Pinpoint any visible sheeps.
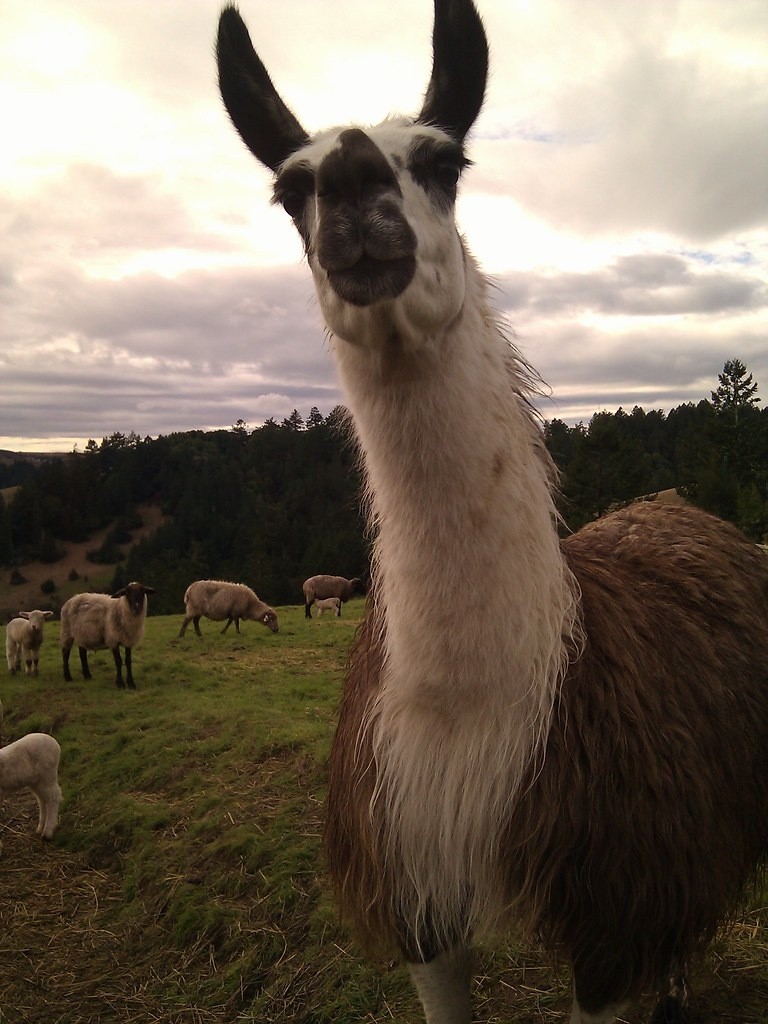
[60,581,157,690]
[178,580,280,638]
[0,733,62,842]
[304,575,365,618]
[6,610,54,676]
